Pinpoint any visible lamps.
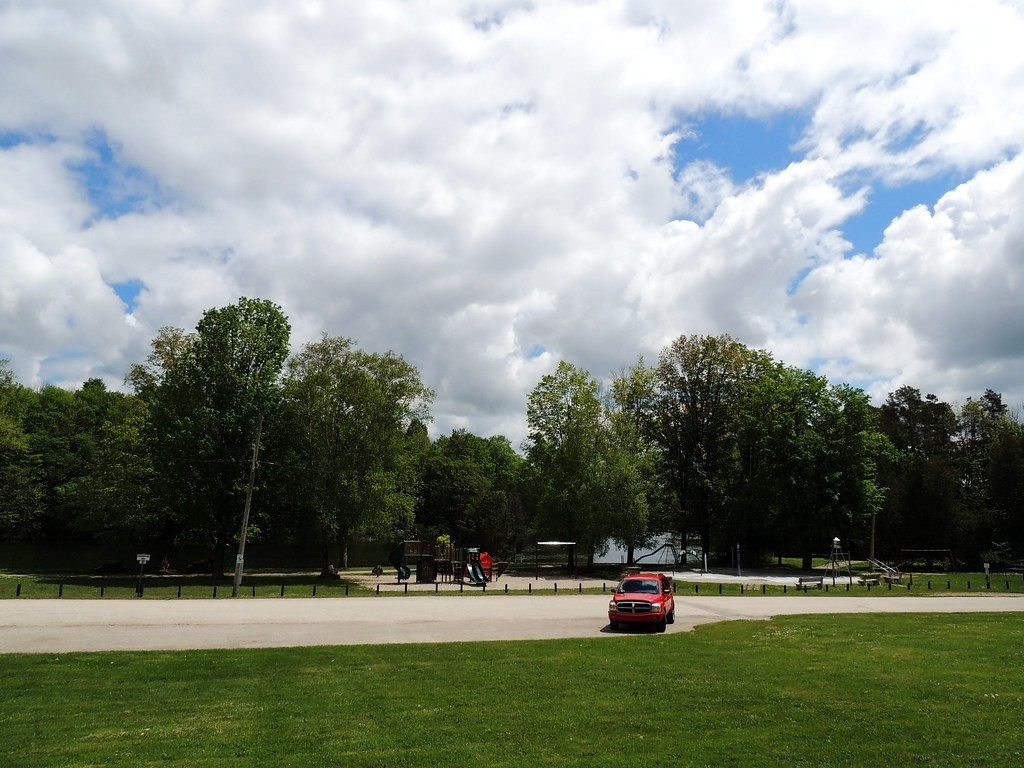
[984,563,990,568]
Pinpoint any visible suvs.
[608,573,675,632]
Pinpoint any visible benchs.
[884,572,904,583]
[858,574,881,586]
[536,564,578,580]
[794,576,824,590]
